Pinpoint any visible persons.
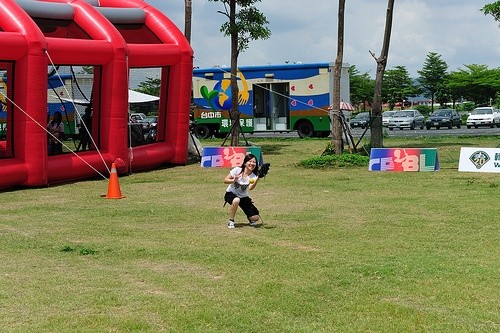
[224,154,270,228]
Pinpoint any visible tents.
[51,88,160,148]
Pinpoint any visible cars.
[466,107,500,128]
[350,112,371,129]
[379,111,397,127]
[139,116,157,132]
[426,109,462,129]
[388,110,425,131]
[131,113,146,121]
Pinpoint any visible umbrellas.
[328,99,355,111]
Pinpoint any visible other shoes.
[247,217,256,225]
[227,221,235,229]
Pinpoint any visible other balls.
[249,178,254,184]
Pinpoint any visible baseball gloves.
[257,163,271,178]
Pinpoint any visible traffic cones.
[101,163,126,199]
